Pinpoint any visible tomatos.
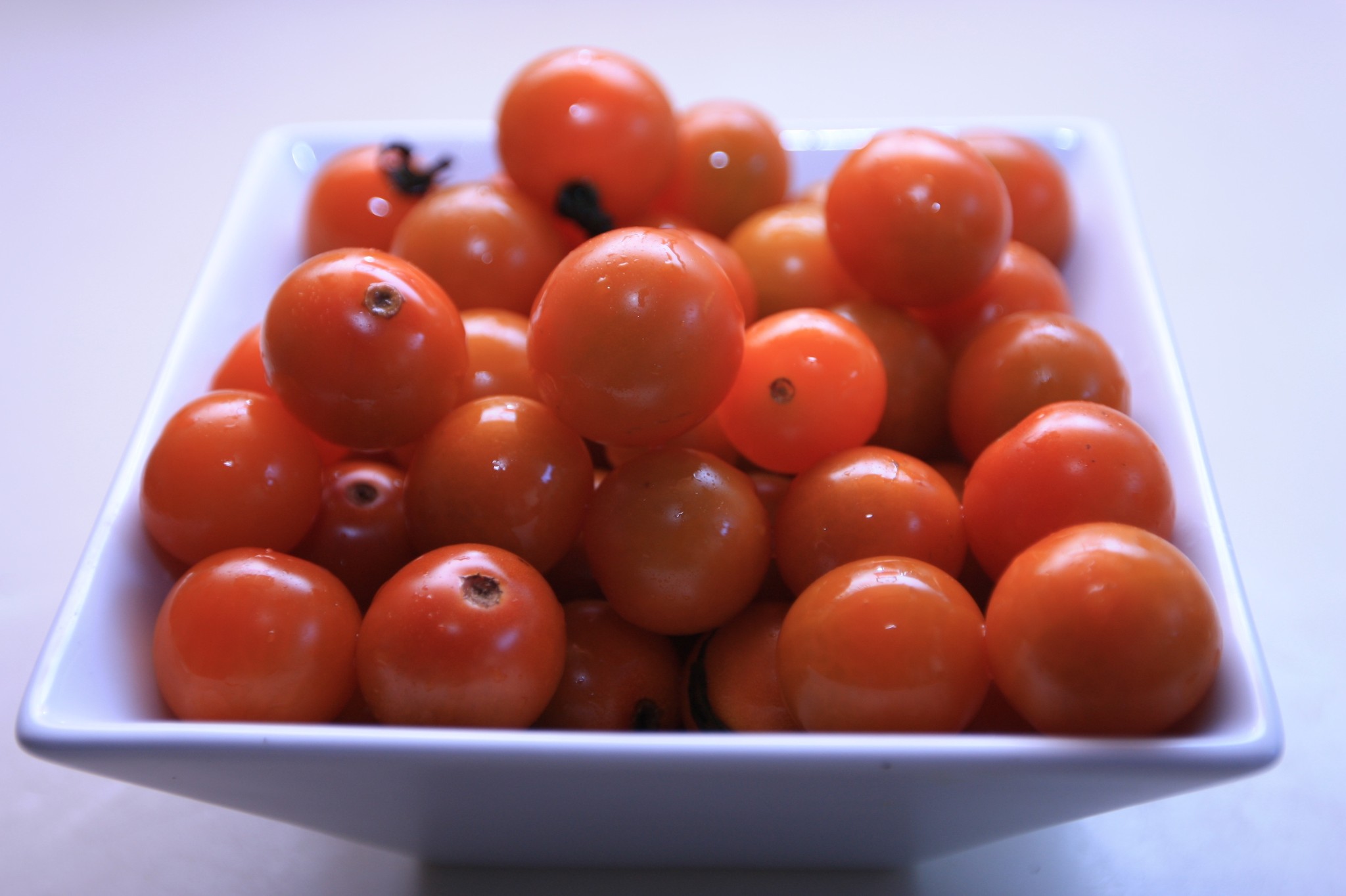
[137,43,1225,741]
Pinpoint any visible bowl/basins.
[14,119,1286,876]
